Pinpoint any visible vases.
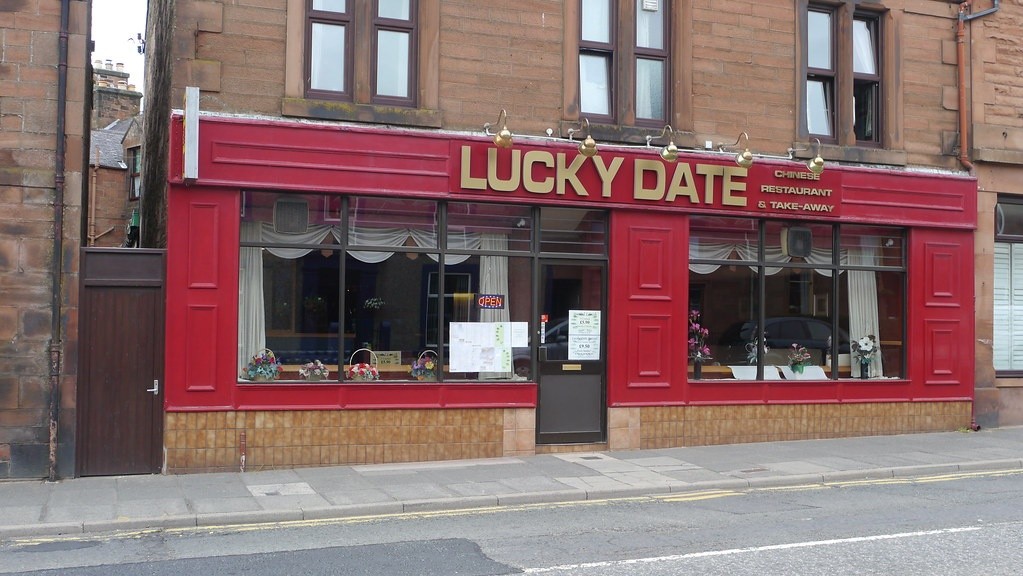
[694,361,701,380]
[861,362,869,379]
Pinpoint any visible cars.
[511,320,570,378]
[719,318,851,364]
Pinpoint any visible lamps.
[567,118,598,157]
[789,138,824,174]
[717,133,753,169]
[484,109,514,149]
[646,125,678,162]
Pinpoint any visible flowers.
[345,363,380,381]
[688,310,714,364]
[849,335,878,364]
[746,337,770,364]
[244,352,283,380]
[408,352,437,380]
[787,343,811,374]
[299,360,329,381]
